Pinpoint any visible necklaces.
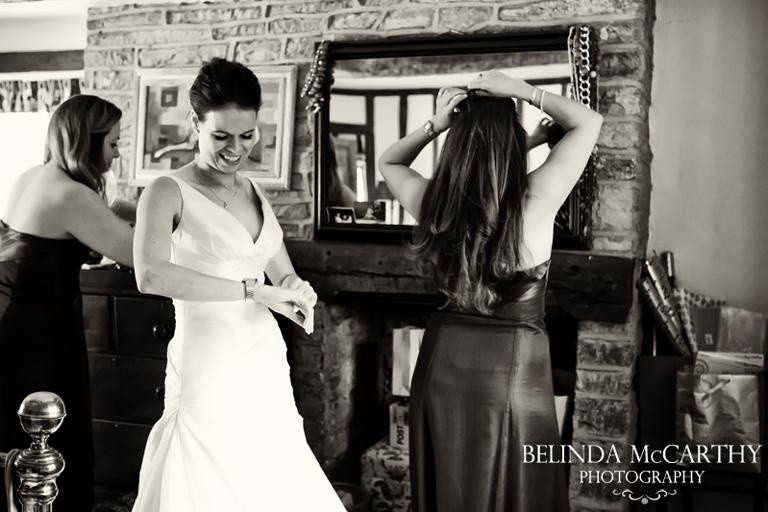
[194,163,240,209]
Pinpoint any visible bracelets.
[528,86,538,105]
[539,88,546,113]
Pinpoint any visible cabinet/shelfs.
[75,263,175,494]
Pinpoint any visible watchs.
[242,277,259,303]
[424,120,439,140]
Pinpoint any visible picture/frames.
[127,64,298,192]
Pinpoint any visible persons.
[0,94,134,511]
[134,58,349,511]
[377,72,606,511]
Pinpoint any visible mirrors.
[311,23,597,253]
